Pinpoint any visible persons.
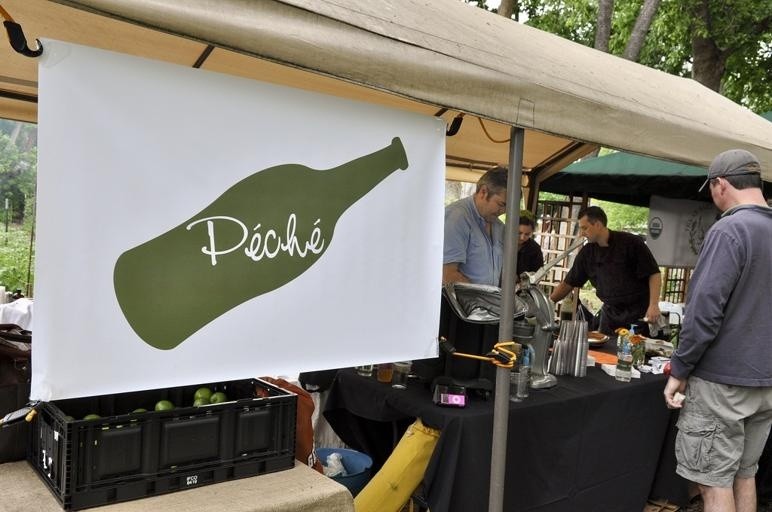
[663,148,772,512]
[548,206,663,340]
[440,165,522,287]
[516,216,544,285]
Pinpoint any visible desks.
[322,341,702,512]
[0,457,354,512]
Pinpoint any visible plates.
[587,331,610,344]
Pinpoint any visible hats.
[698,149,760,193]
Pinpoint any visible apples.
[66,388,226,430]
[619,328,630,336]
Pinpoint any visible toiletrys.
[622,324,639,353]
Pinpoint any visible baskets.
[26,379,299,512]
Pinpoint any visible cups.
[548,320,588,378]
[391,361,413,390]
[509,366,533,404]
[376,363,394,384]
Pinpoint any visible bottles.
[614,342,634,382]
